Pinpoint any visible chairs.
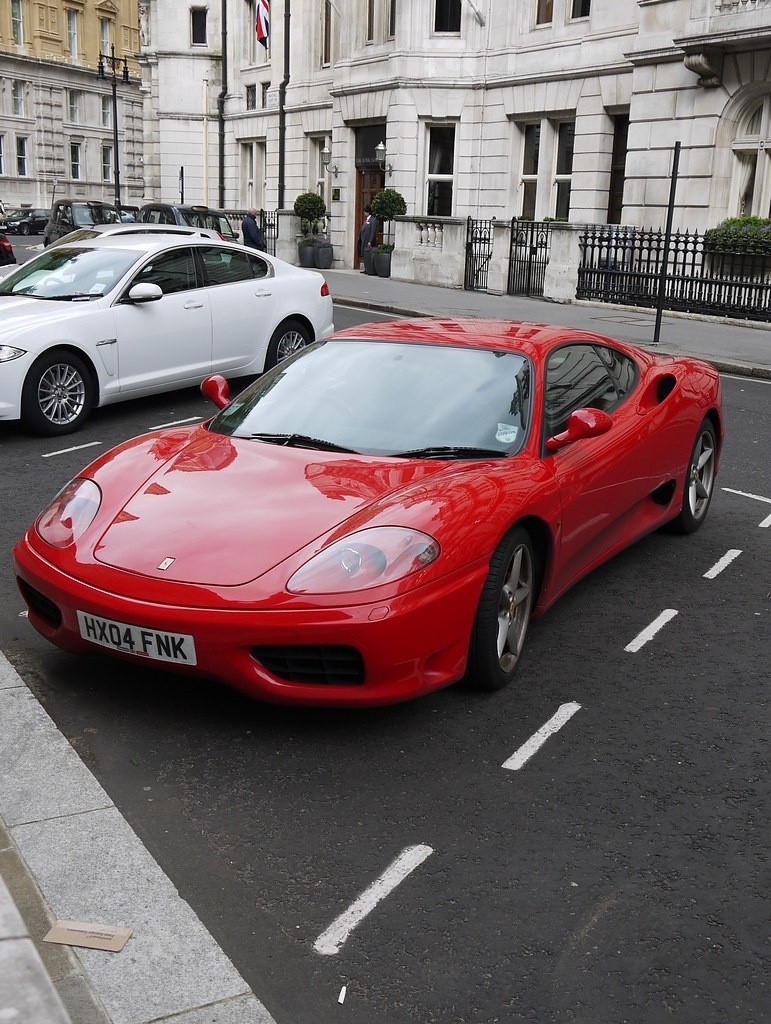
[224,255,251,284]
[204,252,226,283]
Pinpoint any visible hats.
[363,205,372,211]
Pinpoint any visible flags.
[256,0,269,50]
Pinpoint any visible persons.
[358,207,377,274]
[242,208,264,252]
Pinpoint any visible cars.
[43,200,123,247]
[0,223,232,294]
[135,203,240,245]
[0,209,51,236]
[106,210,135,223]
[0,233,334,438]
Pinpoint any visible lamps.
[320,147,338,178]
[374,140,392,177]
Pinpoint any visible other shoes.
[360,270,367,274]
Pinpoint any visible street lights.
[96,43,132,206]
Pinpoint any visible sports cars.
[13,316,725,709]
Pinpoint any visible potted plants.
[363,246,379,275]
[373,243,394,278]
[313,241,333,269]
[298,237,320,268]
